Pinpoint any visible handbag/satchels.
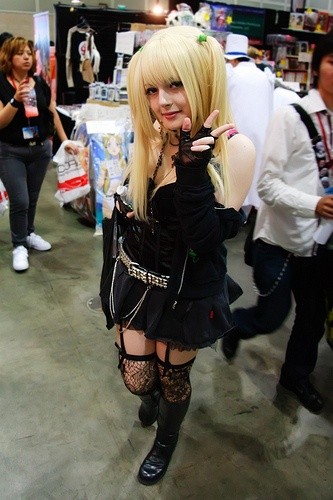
[53,139,91,203]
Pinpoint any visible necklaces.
[151,129,180,182]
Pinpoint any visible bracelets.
[9,97,21,108]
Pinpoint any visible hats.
[224,34,255,60]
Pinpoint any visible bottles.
[21,79,38,118]
[117,184,133,210]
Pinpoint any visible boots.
[139,427,181,486]
[138,398,159,427]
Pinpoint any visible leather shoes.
[279,373,324,413]
[221,307,240,360]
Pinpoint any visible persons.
[100,25,255,484]
[0,37,77,272]
[0,31,13,51]
[205,34,333,412]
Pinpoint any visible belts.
[119,242,169,289]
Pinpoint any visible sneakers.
[12,245,30,271]
[26,232,52,251]
